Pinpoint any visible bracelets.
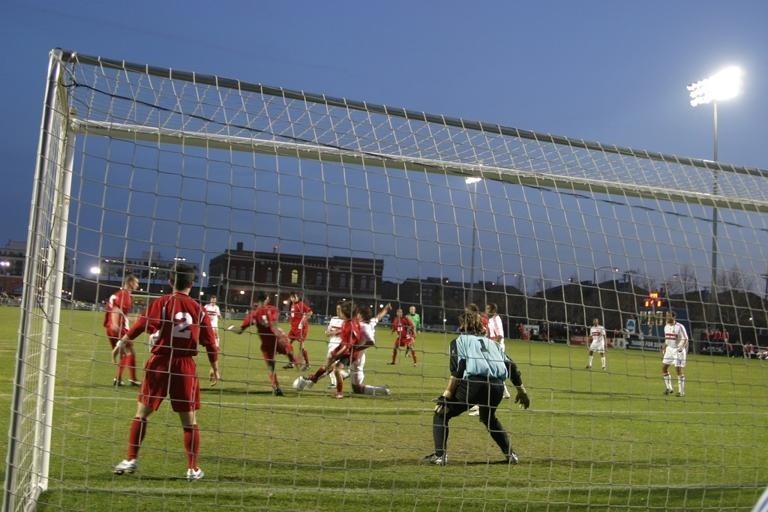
[115,339,127,349]
[514,384,527,394]
[441,389,454,400]
[227,325,235,330]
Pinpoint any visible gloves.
[432,395,451,415]
[515,393,530,409]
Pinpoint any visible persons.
[355,302,392,398]
[280,289,314,372]
[584,317,607,371]
[324,301,350,390]
[699,326,768,360]
[419,312,531,466]
[298,300,363,399]
[102,274,142,389]
[402,305,421,362]
[659,310,689,397]
[386,307,418,368]
[225,291,303,398]
[484,302,511,400]
[465,303,489,418]
[110,260,221,484]
[205,295,224,351]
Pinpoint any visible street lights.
[686,66,746,287]
[594,265,618,321]
[465,160,487,308]
[494,272,519,302]
[672,272,699,293]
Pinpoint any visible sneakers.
[423,454,447,464]
[113,378,141,386]
[283,363,295,368]
[664,389,685,397]
[187,467,204,481]
[507,453,518,464]
[301,375,350,400]
[469,406,479,416]
[302,366,311,371]
[274,388,283,396]
[383,385,391,397]
[114,459,136,474]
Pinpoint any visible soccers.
[292,378,305,390]
